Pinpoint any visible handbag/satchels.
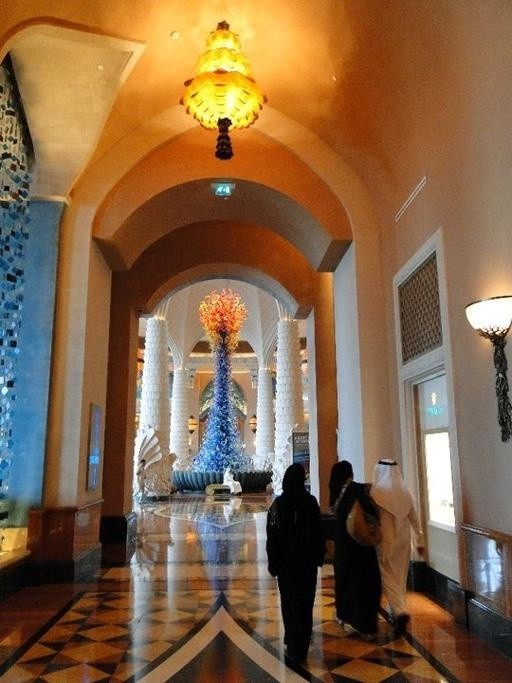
[346,483,383,546]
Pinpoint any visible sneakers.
[394,614,409,638]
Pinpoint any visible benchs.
[205,483,243,498]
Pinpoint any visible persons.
[265,462,326,663]
[134,458,147,505]
[327,460,381,641]
[369,456,425,635]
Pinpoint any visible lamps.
[178,19,269,162]
[464,293,512,443]
[248,414,257,432]
[188,414,197,434]
